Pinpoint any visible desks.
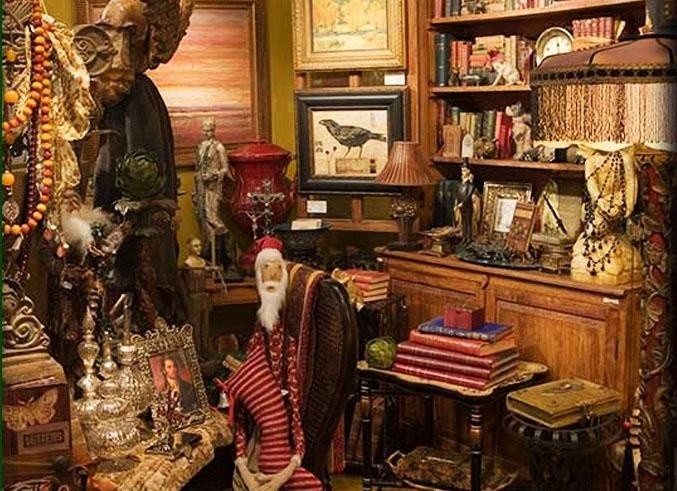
[95,411,234,491]
[354,358,548,491]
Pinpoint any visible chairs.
[179,277,360,490]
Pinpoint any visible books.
[342,268,391,302]
[345,393,385,461]
[435,33,532,86]
[571,16,629,43]
[506,375,625,430]
[434,0,555,18]
[391,315,521,391]
[450,105,513,159]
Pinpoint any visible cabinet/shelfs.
[382,0,677,491]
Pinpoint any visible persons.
[159,353,196,412]
[252,237,290,331]
[191,117,230,239]
[453,156,476,246]
[183,237,214,356]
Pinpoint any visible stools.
[502,412,623,491]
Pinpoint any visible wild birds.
[318,118,387,158]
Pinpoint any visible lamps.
[375,140,439,252]
[530,27,677,489]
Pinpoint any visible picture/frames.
[290,0,409,74]
[75,0,272,168]
[130,317,212,436]
[292,85,411,197]
[479,179,533,236]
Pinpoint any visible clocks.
[535,26,575,67]
